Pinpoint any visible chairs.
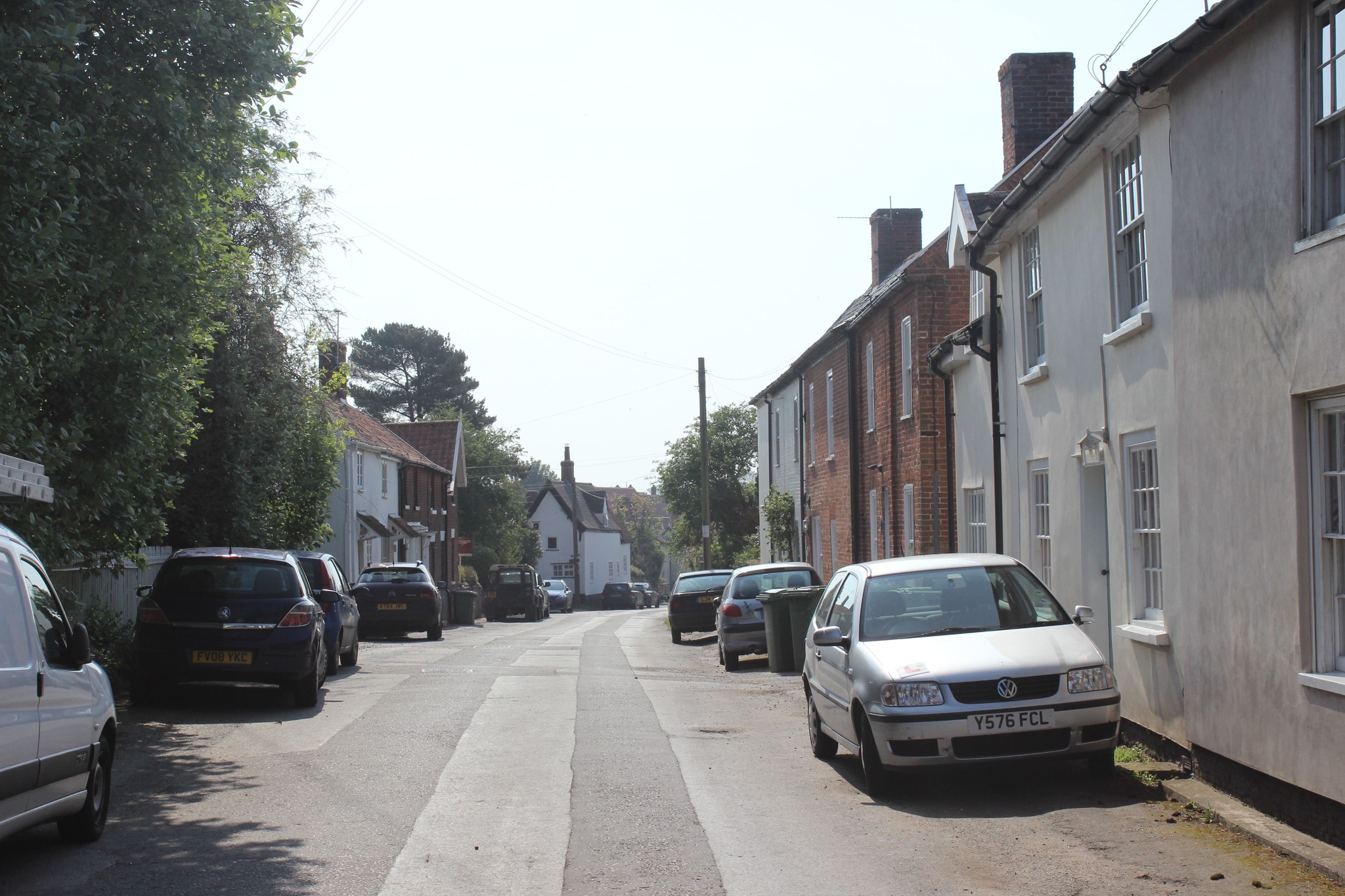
[862,591,908,637]
[739,581,761,599]
[787,574,807,590]
[954,581,1000,632]
[252,569,285,598]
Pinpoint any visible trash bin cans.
[451,589,482,626]
[754,587,795,672]
[783,585,829,675]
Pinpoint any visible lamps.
[1079,426,1109,468]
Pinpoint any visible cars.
[660,569,735,643]
[711,561,825,672]
[801,551,1123,796]
[542,579,573,613]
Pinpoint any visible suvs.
[276,549,371,676]
[633,582,660,608]
[131,546,329,708]
[484,563,542,622]
[499,573,551,618]
[348,559,446,641]
[600,581,644,609]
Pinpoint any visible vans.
[0,521,117,841]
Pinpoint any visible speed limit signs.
[696,553,704,564]
